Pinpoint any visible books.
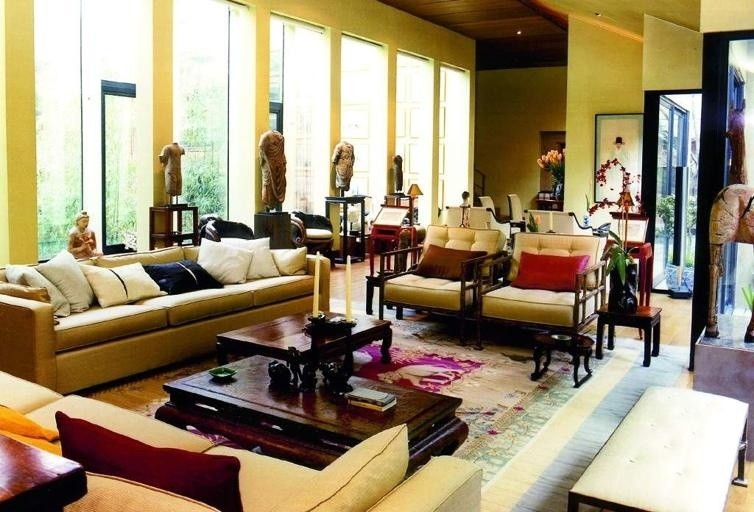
[348,387,397,412]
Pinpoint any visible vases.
[553,179,564,201]
[608,270,638,315]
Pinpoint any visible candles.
[312,250,320,318]
[345,255,352,323]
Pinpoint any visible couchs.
[378,227,607,336]
[1,248,330,395]
[0,371,483,511]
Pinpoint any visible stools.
[530,333,595,388]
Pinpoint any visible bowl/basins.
[208,367,237,382]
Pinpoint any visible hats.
[616,137,622,143]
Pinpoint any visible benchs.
[568,384,748,512]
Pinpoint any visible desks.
[595,303,662,369]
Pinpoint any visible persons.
[67,211,97,258]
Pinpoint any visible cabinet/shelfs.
[150,193,413,268]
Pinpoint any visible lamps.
[407,184,424,196]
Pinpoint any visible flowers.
[537,146,565,183]
[588,159,646,287]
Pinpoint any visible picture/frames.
[594,113,644,203]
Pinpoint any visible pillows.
[57,408,245,511]
[0,402,59,443]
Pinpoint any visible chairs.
[445,194,576,242]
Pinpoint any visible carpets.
[95,297,693,512]
